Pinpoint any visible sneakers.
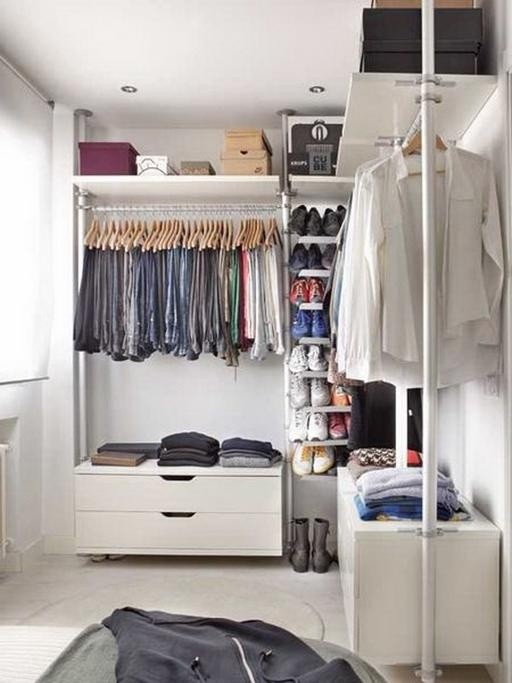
[289,345,328,374]
[291,242,336,270]
[289,205,346,236]
[290,413,352,442]
[291,310,328,338]
[292,445,349,476]
[291,377,353,408]
[290,277,325,305]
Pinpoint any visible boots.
[312,518,331,572]
[290,518,309,573]
